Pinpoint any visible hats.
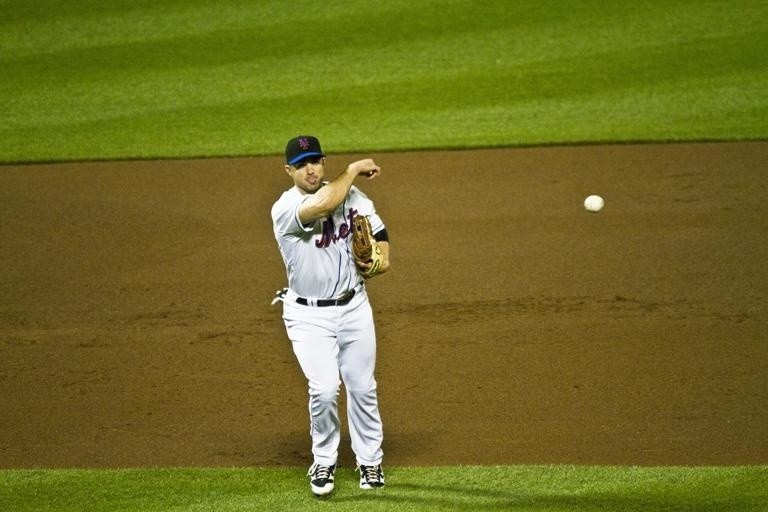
[285,135,324,166]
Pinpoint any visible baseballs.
[584,195,603,211]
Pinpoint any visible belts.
[297,281,366,306]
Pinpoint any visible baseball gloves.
[351,215,383,280]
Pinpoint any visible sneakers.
[355,460,385,491]
[308,460,338,496]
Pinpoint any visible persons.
[270,135,390,496]
